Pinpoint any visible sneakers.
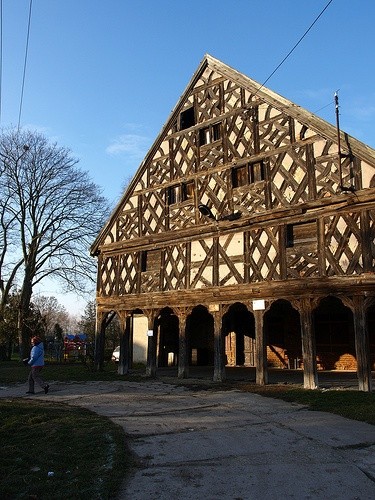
[25,391,34,394]
[44,385,50,394]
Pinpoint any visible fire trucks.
[63,334,87,363]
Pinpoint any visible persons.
[23,335,50,395]
